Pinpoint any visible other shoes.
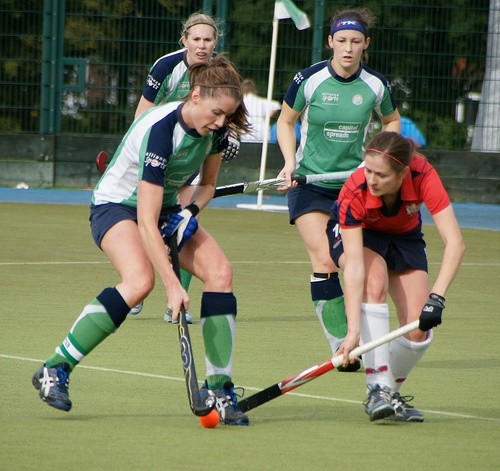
[128,300,143,315]
[335,346,360,373]
[164,308,194,324]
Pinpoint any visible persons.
[275,7,401,372]
[325,132,465,423]
[32,59,251,426]
[269,120,301,143]
[238,79,281,143]
[129,12,240,323]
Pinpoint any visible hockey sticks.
[200,319,420,413]
[95,151,358,199]
[168,229,217,416]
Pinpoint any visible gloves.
[162,203,199,256]
[419,293,445,332]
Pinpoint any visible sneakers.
[384,392,424,422]
[363,383,394,422]
[199,380,249,426]
[32,361,72,412]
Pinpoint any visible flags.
[274,0,310,30]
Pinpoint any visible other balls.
[200,409,220,429]
